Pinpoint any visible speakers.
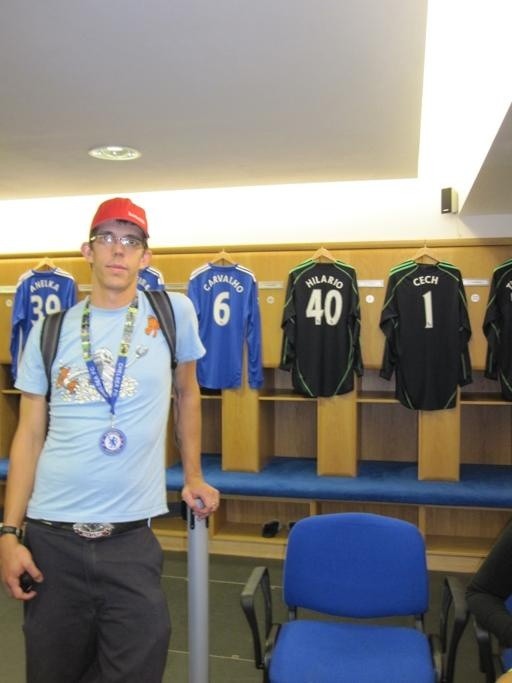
[440,187,458,214]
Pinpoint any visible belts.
[42,515,148,538]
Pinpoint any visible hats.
[89,196,147,237]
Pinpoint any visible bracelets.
[0,522,22,539]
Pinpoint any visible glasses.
[89,233,142,248]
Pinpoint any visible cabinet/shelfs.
[0,236,510,575]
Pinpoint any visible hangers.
[18,255,76,285]
[187,244,461,284]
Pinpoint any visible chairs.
[241,512,472,683]
[449,512,511,683]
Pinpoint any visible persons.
[0,196,219,683]
[465,518,511,682]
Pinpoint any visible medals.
[97,425,129,455]
